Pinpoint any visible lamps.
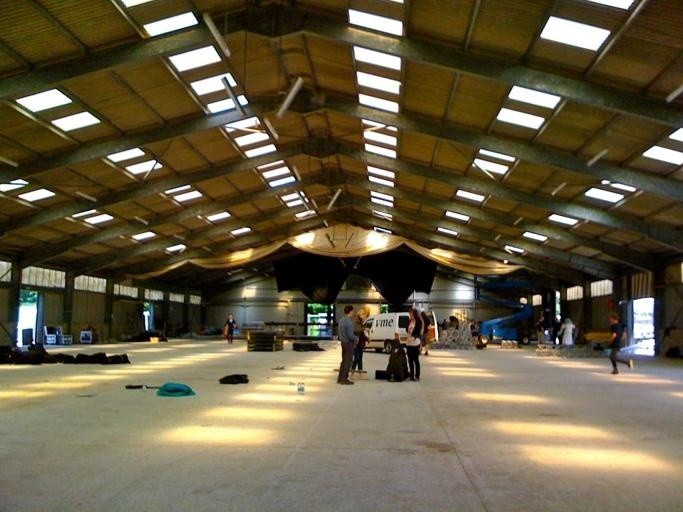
[201,11,307,140]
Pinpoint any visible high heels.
[408,375,421,381]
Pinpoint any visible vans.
[362,311,439,353]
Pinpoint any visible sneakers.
[351,370,369,380]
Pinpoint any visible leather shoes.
[337,378,355,385]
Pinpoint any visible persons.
[536,316,545,346]
[336,305,357,384]
[405,308,424,382]
[351,309,371,373]
[468,318,476,338]
[557,317,576,359]
[419,311,430,355]
[550,318,562,349]
[608,313,634,375]
[441,319,449,330]
[224,314,237,345]
